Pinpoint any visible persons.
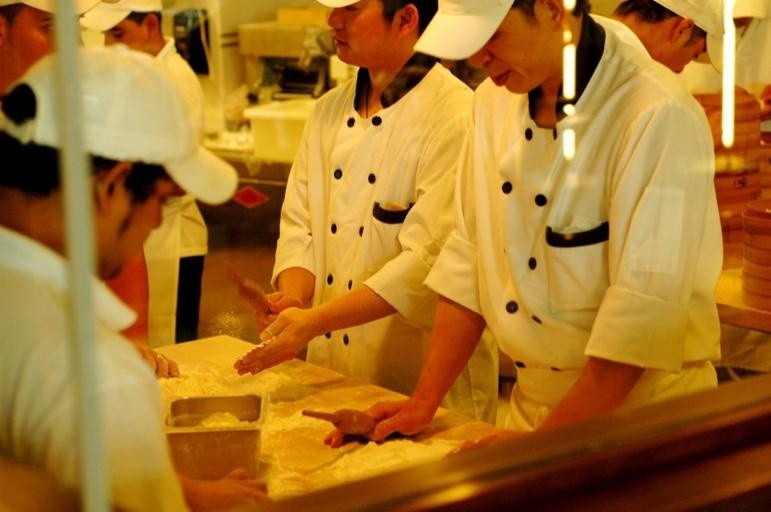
[232,0,498,427]
[1,1,182,381]
[0,40,275,512]
[79,0,210,345]
[611,0,722,74]
[324,0,724,462]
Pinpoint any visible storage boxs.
[243,97,319,161]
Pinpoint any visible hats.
[78,0,166,32]
[1,0,102,20]
[411,1,517,62]
[1,42,243,210]
[653,1,726,76]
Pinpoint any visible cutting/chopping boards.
[153,334,502,500]
